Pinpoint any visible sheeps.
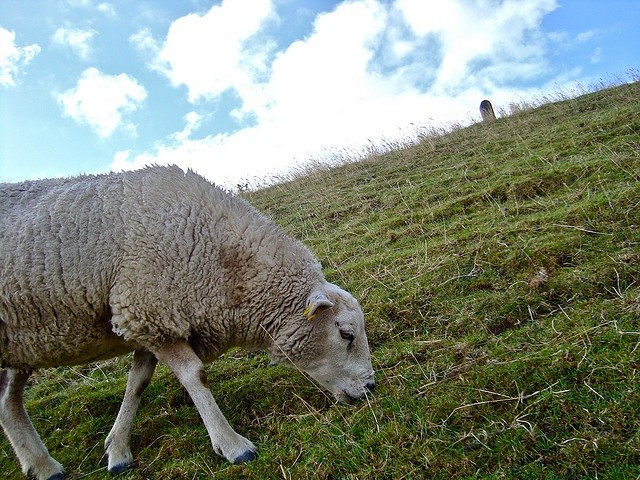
[0,164,376,480]
